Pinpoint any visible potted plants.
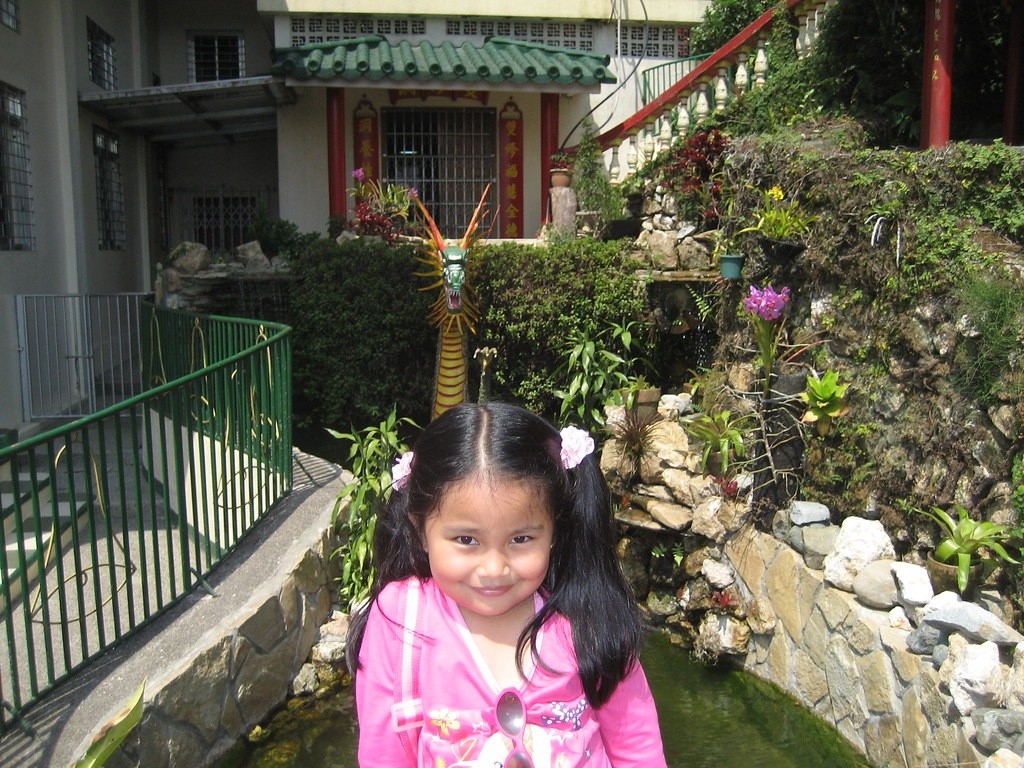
[720,241,745,280]
[736,186,820,268]
[752,362,850,532]
[602,372,661,425]
[912,503,1023,601]
[680,411,759,481]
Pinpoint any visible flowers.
[560,426,595,470]
[550,153,573,168]
[392,451,414,491]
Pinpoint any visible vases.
[550,168,569,187]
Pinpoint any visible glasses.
[495,686,535,768]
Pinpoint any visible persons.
[356,402,669,768]
